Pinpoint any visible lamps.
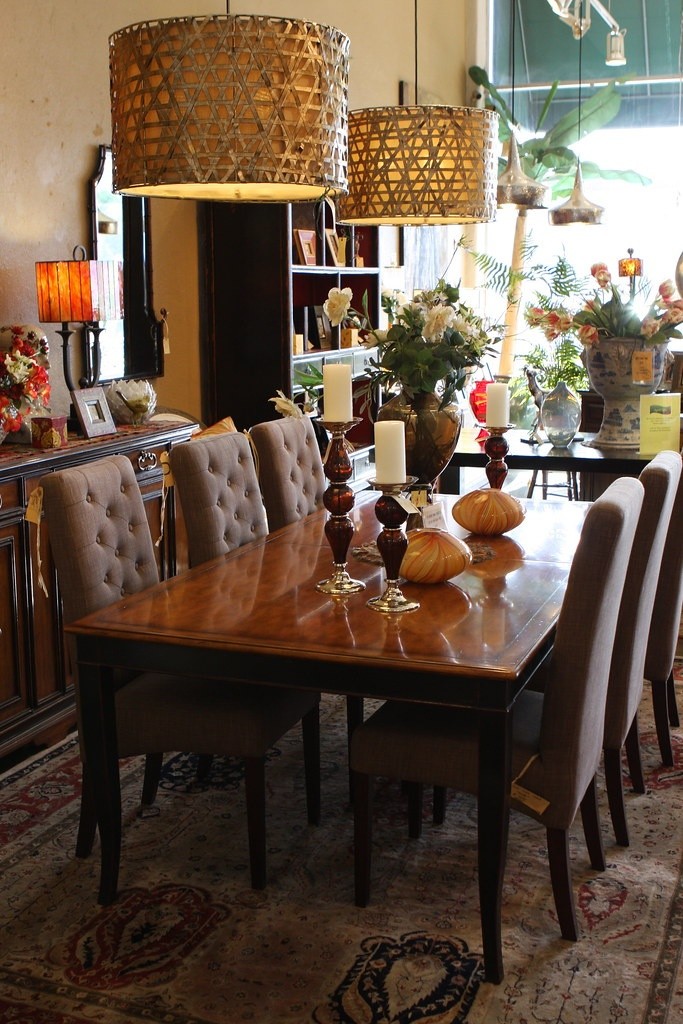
[108,0,350,203]
[337,0,501,227]
[547,0,627,66]
[549,0,604,226]
[496,0,550,211]
[618,248,643,296]
[35,244,124,432]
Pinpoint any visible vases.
[586,335,670,449]
[540,383,581,448]
[0,398,19,444]
[378,384,463,505]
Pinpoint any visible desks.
[62,493,596,984]
[368,425,683,501]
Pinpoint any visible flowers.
[526,263,683,349]
[0,322,52,432]
[324,230,535,467]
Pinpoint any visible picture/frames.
[297,306,332,353]
[71,386,118,438]
[293,229,317,266]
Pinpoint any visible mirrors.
[86,146,168,388]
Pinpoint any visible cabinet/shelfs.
[0,421,199,776]
[215,200,384,489]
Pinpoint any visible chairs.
[250,416,326,531]
[168,433,369,800]
[353,451,682,940]
[38,456,320,893]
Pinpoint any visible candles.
[322,362,353,421]
[373,421,406,485]
[487,383,510,428]
